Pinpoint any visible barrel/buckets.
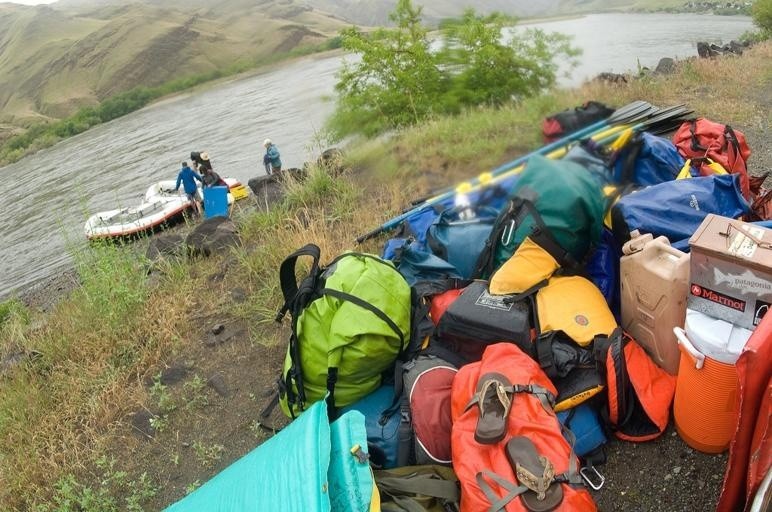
[202,185,228,222]
[617,228,692,377]
[446,215,496,279]
[672,309,753,457]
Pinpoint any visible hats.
[200,152,208,160]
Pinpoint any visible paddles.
[356,100,696,243]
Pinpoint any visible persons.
[263,139,282,173]
[190,151,212,174]
[198,166,231,194]
[174,162,203,216]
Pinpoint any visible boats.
[83,198,195,245]
[143,176,250,210]
